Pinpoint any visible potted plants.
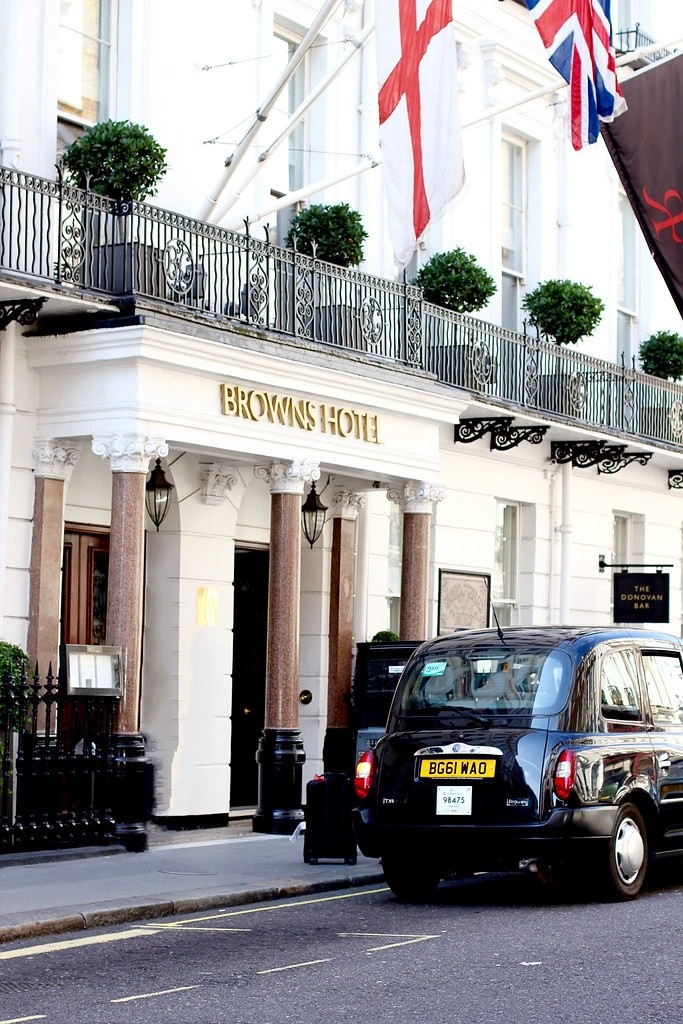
[286,203,367,353]
[638,330,683,444]
[59,118,168,301]
[409,248,492,391]
[520,277,604,419]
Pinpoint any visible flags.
[372,0,465,275]
[525,0,628,151]
[598,51,683,319]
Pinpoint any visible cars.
[353,624,683,900]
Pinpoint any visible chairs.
[419,666,543,701]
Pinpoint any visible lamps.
[144,458,175,532]
[300,474,331,549]
